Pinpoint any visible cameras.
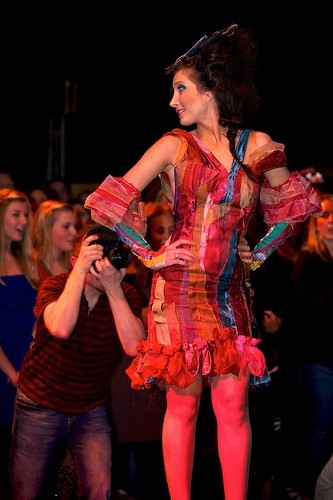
[89,233,132,273]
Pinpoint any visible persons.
[83,25,322,500]
[11,226,145,500]
[0,170,333,500]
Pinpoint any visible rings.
[175,253,179,258]
[248,253,253,258]
